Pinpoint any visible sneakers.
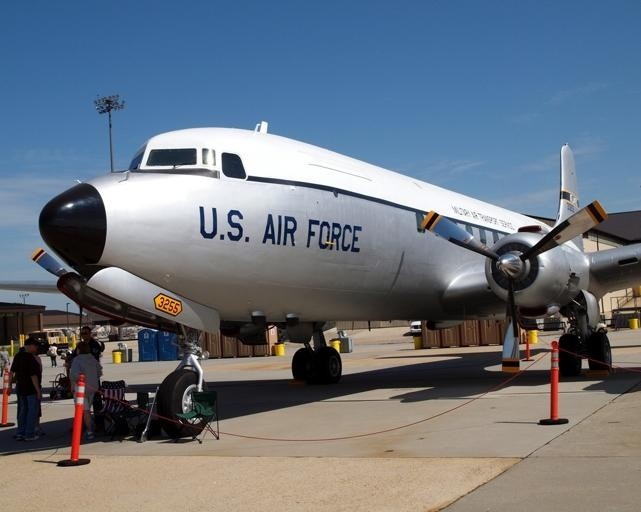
[82,433,95,440]
[15,429,45,441]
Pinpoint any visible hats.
[25,338,40,345]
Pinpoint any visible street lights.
[90,94,126,175]
[65,301,70,330]
[17,293,29,305]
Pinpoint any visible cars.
[25,328,68,353]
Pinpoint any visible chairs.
[176,391,219,444]
[98,380,127,435]
[109,392,149,439]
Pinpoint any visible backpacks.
[50,350,57,357]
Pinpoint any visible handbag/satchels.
[47,351,50,356]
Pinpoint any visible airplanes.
[27,113,641,439]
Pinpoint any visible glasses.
[81,332,89,335]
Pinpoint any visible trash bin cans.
[329,339,341,354]
[528,330,537,343]
[112,350,122,363]
[628,318,638,329]
[274,343,285,356]
[413,332,422,349]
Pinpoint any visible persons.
[64,326,105,440]
[7,339,43,441]
[0,345,10,377]
[48,343,58,368]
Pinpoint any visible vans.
[408,320,421,335]
